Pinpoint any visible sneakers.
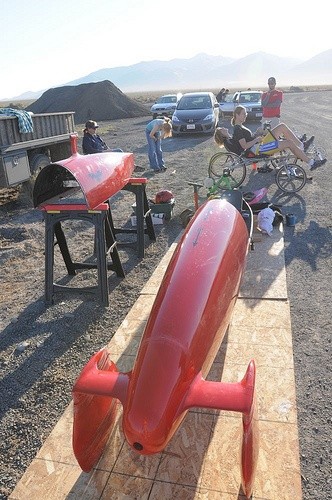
[303,136,315,152]
[310,159,327,170]
[301,133,307,142]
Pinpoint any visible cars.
[150,94,181,119]
[232,90,267,122]
[171,92,221,137]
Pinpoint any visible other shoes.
[154,167,161,171]
[160,165,167,170]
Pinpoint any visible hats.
[268,77,276,85]
[85,120,99,128]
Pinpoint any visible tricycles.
[206,120,328,195]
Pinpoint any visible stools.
[131,199,176,219]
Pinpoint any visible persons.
[146,117,172,172]
[215,106,327,171]
[82,120,123,154]
[258,77,282,173]
[216,88,229,103]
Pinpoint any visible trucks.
[0,112,77,191]
